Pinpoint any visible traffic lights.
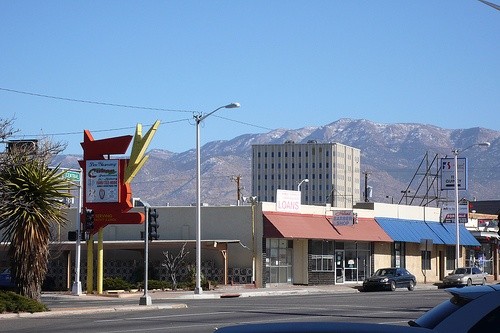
[84,207,94,231]
[68,231,78,241]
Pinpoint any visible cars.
[408,281,500,333]
[363,267,416,292]
[442,267,488,288]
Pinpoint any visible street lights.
[297,178,309,191]
[453,141,491,271]
[194,102,240,294]
